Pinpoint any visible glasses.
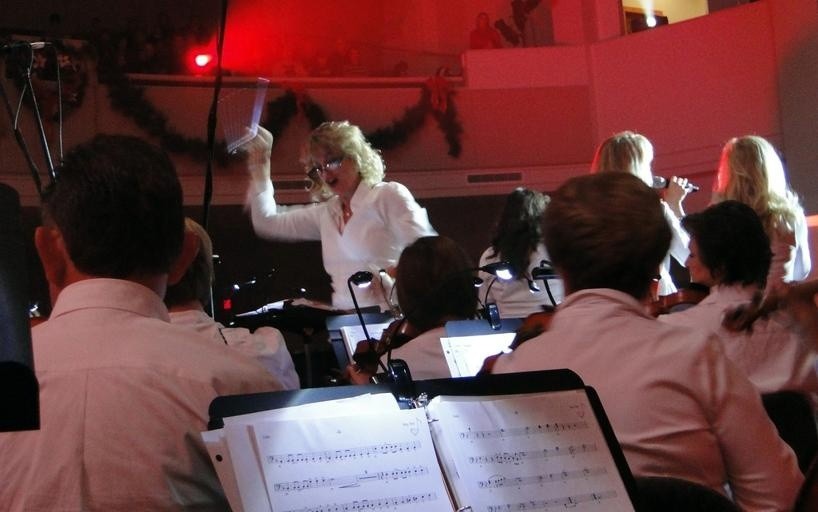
[306,156,344,180]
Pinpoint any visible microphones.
[651,175,702,193]
[2,40,51,54]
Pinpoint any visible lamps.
[222,261,561,382]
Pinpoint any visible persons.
[243,121,438,369]
[346,237,494,382]
[710,135,812,284]
[490,173,804,512]
[1,133,299,511]
[656,201,818,409]
[479,189,564,321]
[469,15,501,49]
[164,216,300,393]
[591,132,694,301]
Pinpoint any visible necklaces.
[340,207,354,219]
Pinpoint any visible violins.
[476,305,555,376]
[650,286,709,319]
[352,318,419,373]
